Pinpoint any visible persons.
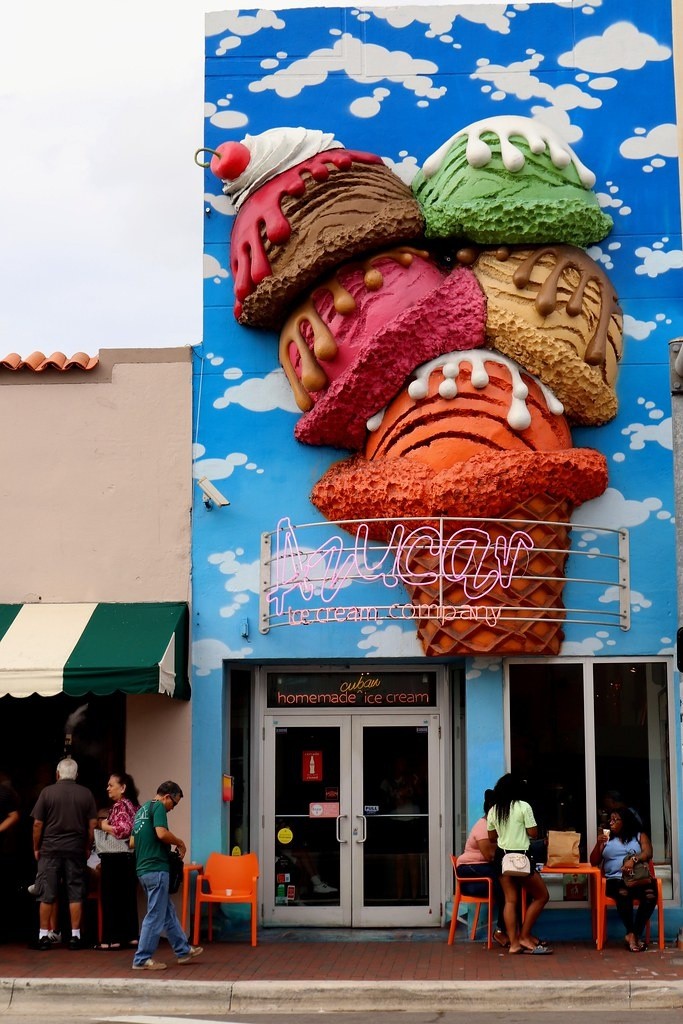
[590,805,658,952]
[0,783,20,832]
[30,758,113,951]
[94,772,142,950]
[487,774,553,955]
[602,783,651,844]
[386,757,424,900]
[456,789,547,947]
[277,817,338,893]
[130,780,204,971]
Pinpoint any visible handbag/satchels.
[623,848,649,882]
[93,801,135,854]
[547,830,580,868]
[502,854,530,876]
[166,850,183,894]
[276,856,294,874]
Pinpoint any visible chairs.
[86,864,107,943]
[193,853,259,947]
[598,860,665,950]
[447,855,520,950]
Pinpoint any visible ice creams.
[195,114,624,656]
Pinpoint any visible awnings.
[0,601,191,701]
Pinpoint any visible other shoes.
[624,935,639,952]
[46,930,62,942]
[125,938,138,949]
[37,935,52,950]
[94,940,121,951]
[68,936,84,950]
[636,940,648,951]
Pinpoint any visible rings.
[628,869,629,870]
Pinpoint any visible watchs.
[632,857,638,863]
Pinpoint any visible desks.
[522,863,607,942]
[181,864,204,933]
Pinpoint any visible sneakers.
[177,946,203,963]
[314,883,338,893]
[131,959,168,970]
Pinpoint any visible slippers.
[509,946,527,955]
[493,934,511,948]
[535,940,547,947]
[523,945,554,954]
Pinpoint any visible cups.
[226,889,232,896]
[603,829,610,844]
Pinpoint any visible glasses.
[168,795,177,806]
[608,817,622,823]
